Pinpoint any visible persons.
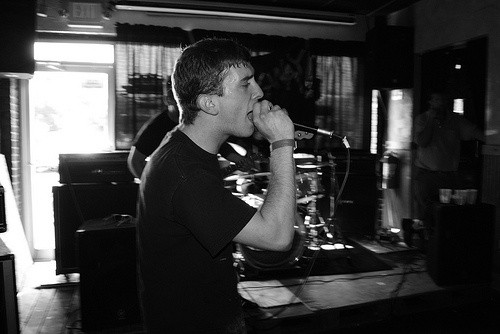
[128,76,180,180]
[137,40,296,334]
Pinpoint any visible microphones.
[291,121,346,141]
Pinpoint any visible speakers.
[54,182,140,274]
[74,215,142,334]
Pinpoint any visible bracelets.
[270,139,297,150]
